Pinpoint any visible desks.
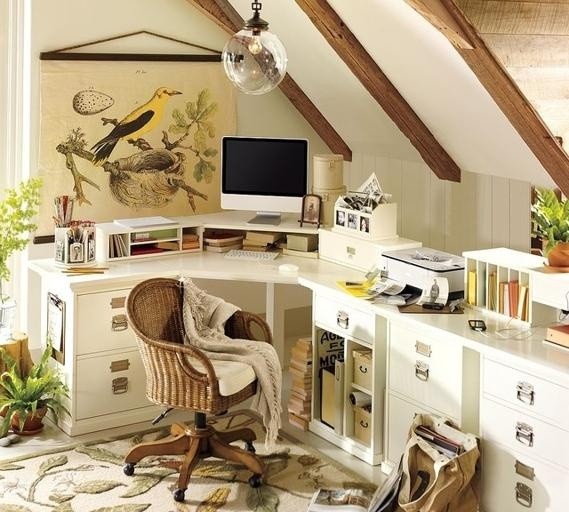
[27,210,568,511]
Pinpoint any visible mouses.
[278,263,300,272]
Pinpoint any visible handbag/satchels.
[395,414,482,512]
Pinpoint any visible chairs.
[123,279,275,500]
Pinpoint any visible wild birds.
[89,87,183,168]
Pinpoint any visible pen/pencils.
[346,282,362,285]
[62,268,109,276]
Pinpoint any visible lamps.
[221,0,289,96]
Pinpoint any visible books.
[542,338,569,351]
[202,244,243,253]
[546,324,569,346]
[308,452,405,512]
[242,238,270,247]
[204,239,243,247]
[107,231,201,258]
[245,231,283,245]
[415,423,465,457]
[282,247,319,258]
[113,216,180,229]
[242,246,275,252]
[288,336,312,432]
[467,269,530,322]
[204,232,244,244]
[46,303,63,352]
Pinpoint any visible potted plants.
[1,337,72,439]
[526,188,569,273]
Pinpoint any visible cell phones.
[468,319,486,331]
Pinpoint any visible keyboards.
[224,249,280,262]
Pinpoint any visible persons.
[337,211,345,227]
[358,217,369,233]
[73,246,81,261]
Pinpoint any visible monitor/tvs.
[220,135,310,225]
